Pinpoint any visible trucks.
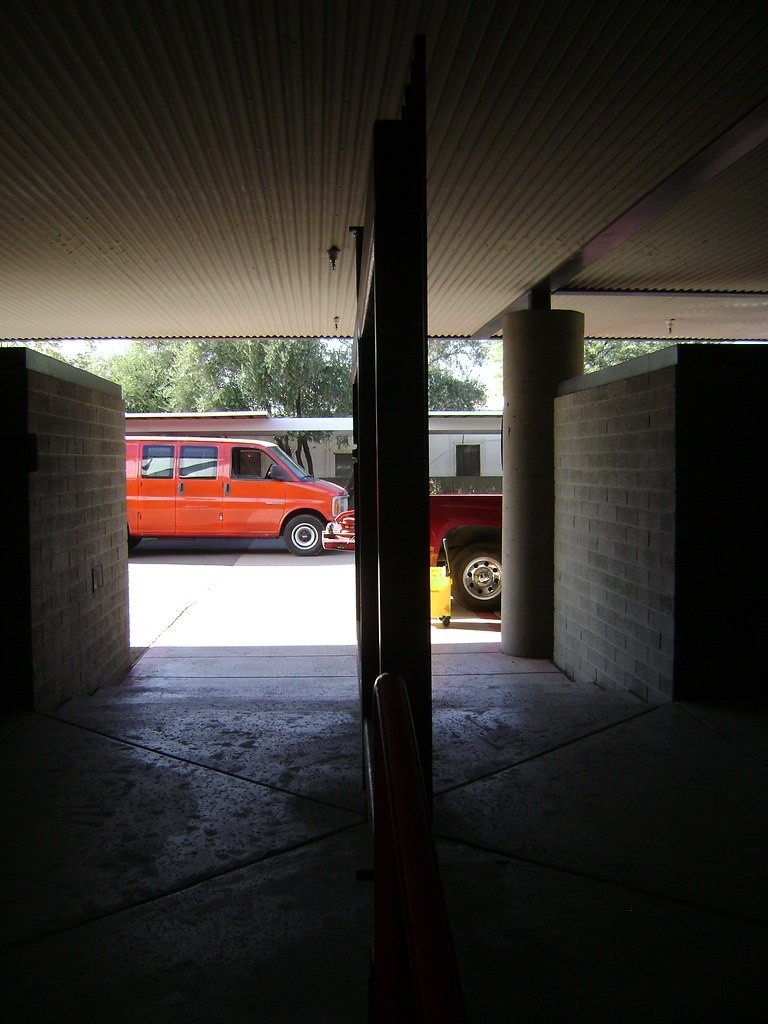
[321,493,504,611]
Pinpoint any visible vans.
[124,436,350,558]
